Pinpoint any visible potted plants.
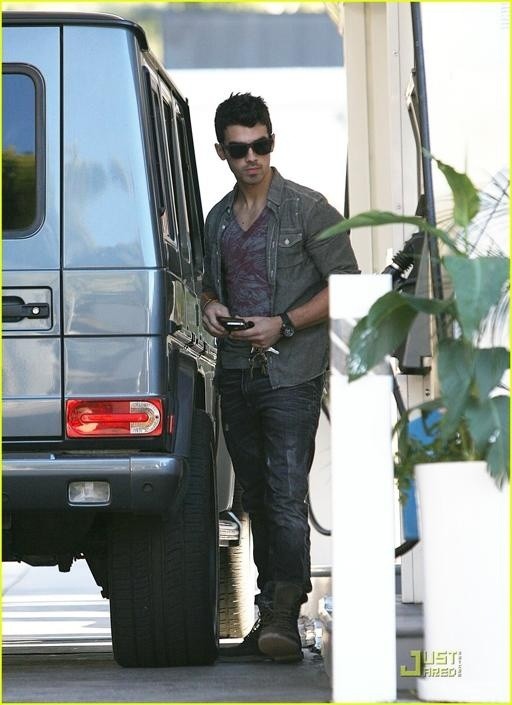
[315,147,508,702]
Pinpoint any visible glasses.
[221,138,274,159]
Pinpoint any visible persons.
[201,92,363,664]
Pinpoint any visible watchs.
[277,313,296,338]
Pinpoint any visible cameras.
[217,317,253,331]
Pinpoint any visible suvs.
[2,12,254,667]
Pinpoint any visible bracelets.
[201,298,218,313]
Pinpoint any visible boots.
[219,580,305,664]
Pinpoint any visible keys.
[248,347,280,368]
